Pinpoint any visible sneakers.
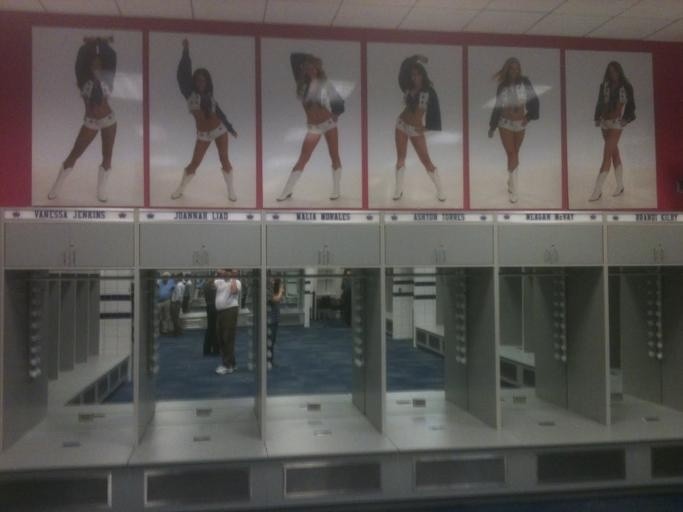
[215,365,237,374]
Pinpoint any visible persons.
[155,268,254,377]
[587,59,638,203]
[45,32,119,203]
[390,52,444,203]
[485,56,541,203]
[275,49,347,201]
[169,35,239,203]
[266,274,287,369]
[339,268,352,327]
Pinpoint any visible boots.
[588,170,608,201]
[172,168,195,199]
[98,165,111,202]
[427,167,446,201]
[276,169,302,201]
[329,167,342,199]
[507,163,518,202]
[393,166,406,199]
[48,164,73,200]
[221,167,237,200]
[613,164,624,196]
